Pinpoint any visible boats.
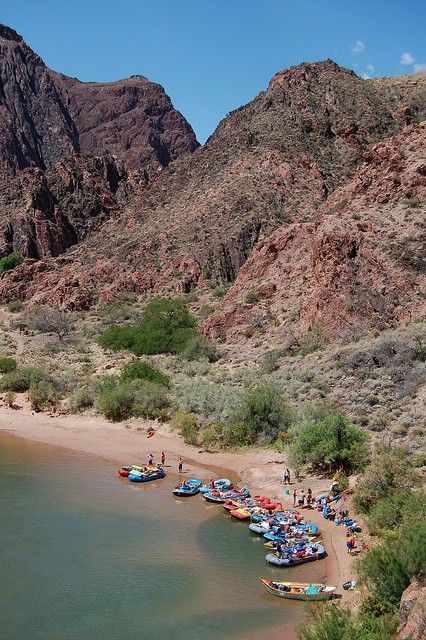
[172,479,201,495]
[231,503,282,519]
[198,479,230,493]
[266,544,325,566]
[263,524,318,540]
[249,516,305,533]
[118,464,153,476]
[264,536,316,549]
[223,496,270,510]
[128,470,163,481]
[258,576,335,600]
[203,489,249,501]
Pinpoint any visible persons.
[178,457,185,474]
[276,542,284,559]
[146,453,154,464]
[161,451,166,467]
[292,489,299,509]
[347,534,356,555]
[283,467,291,485]
[335,512,344,525]
[298,490,305,509]
[326,508,336,520]
[209,477,214,489]
[307,486,312,504]
